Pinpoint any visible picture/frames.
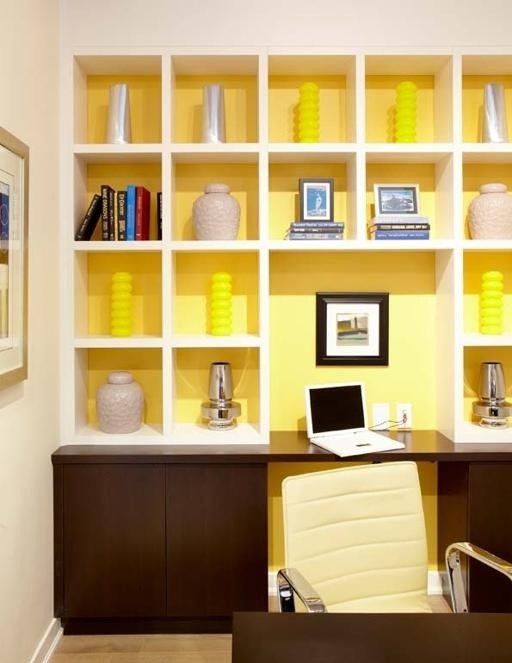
[314,291,390,367]
[0,128,29,390]
[299,178,334,222]
[373,183,420,216]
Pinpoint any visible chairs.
[276,459,512,614]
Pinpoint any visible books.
[285,220,343,240]
[75,183,162,241]
[368,215,430,241]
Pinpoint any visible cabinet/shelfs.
[65,46,512,451]
[435,452,511,615]
[51,444,270,635]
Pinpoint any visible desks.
[230,609,512,662]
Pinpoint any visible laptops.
[304,382,405,458]
